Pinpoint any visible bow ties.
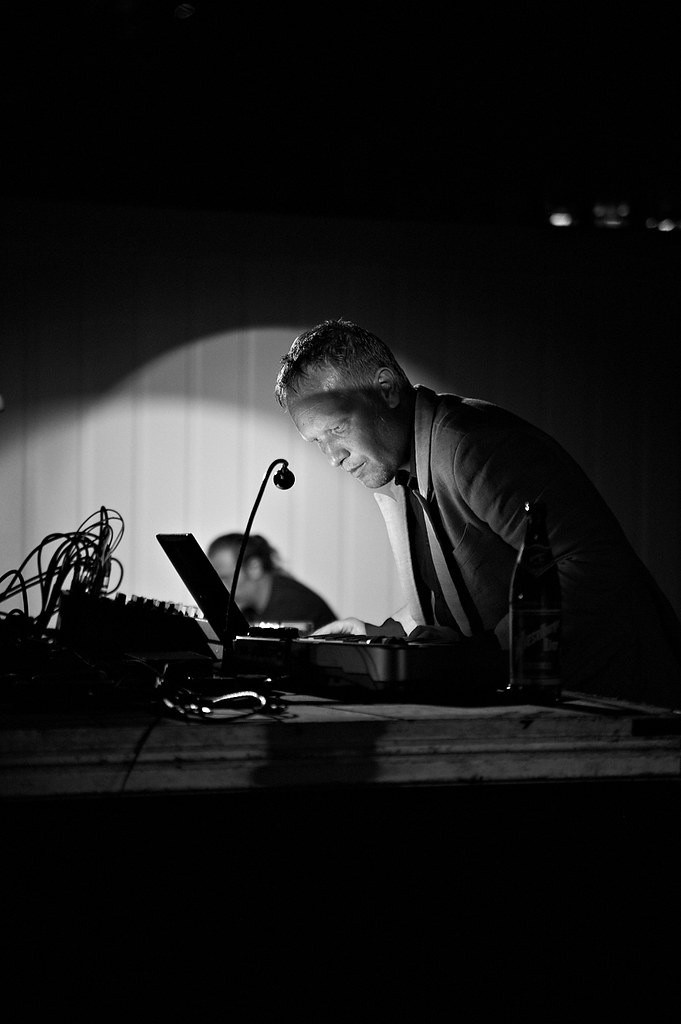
[393,471,417,490]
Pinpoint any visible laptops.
[155,533,249,647]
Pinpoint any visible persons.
[203,530,337,632]
[275,318,611,703]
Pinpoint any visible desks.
[1,689,681,1024]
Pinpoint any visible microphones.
[193,458,295,691]
